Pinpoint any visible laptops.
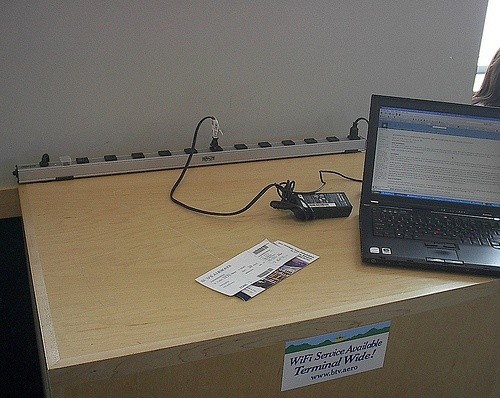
[359,93,500,276]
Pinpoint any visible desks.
[16,148,500,398]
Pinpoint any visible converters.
[292,191,352,219]
